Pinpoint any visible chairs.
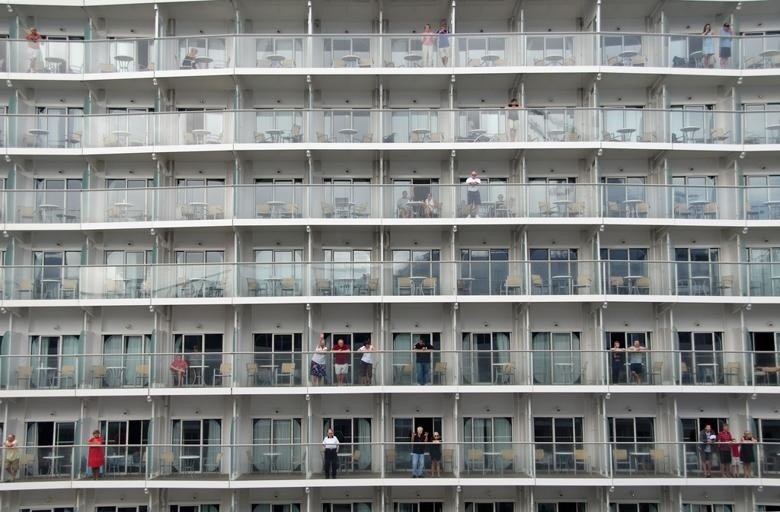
[404,48,780,478]
[1,50,404,479]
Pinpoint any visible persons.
[171,345,201,387]
[411,427,443,478]
[309,331,375,385]
[26,27,41,72]
[397,191,434,218]
[186,47,198,68]
[4,434,20,480]
[700,424,758,478]
[466,171,505,219]
[421,23,450,67]
[322,429,340,479]
[87,429,104,479]
[505,98,520,142]
[701,22,733,68]
[413,338,435,385]
[610,340,648,384]
